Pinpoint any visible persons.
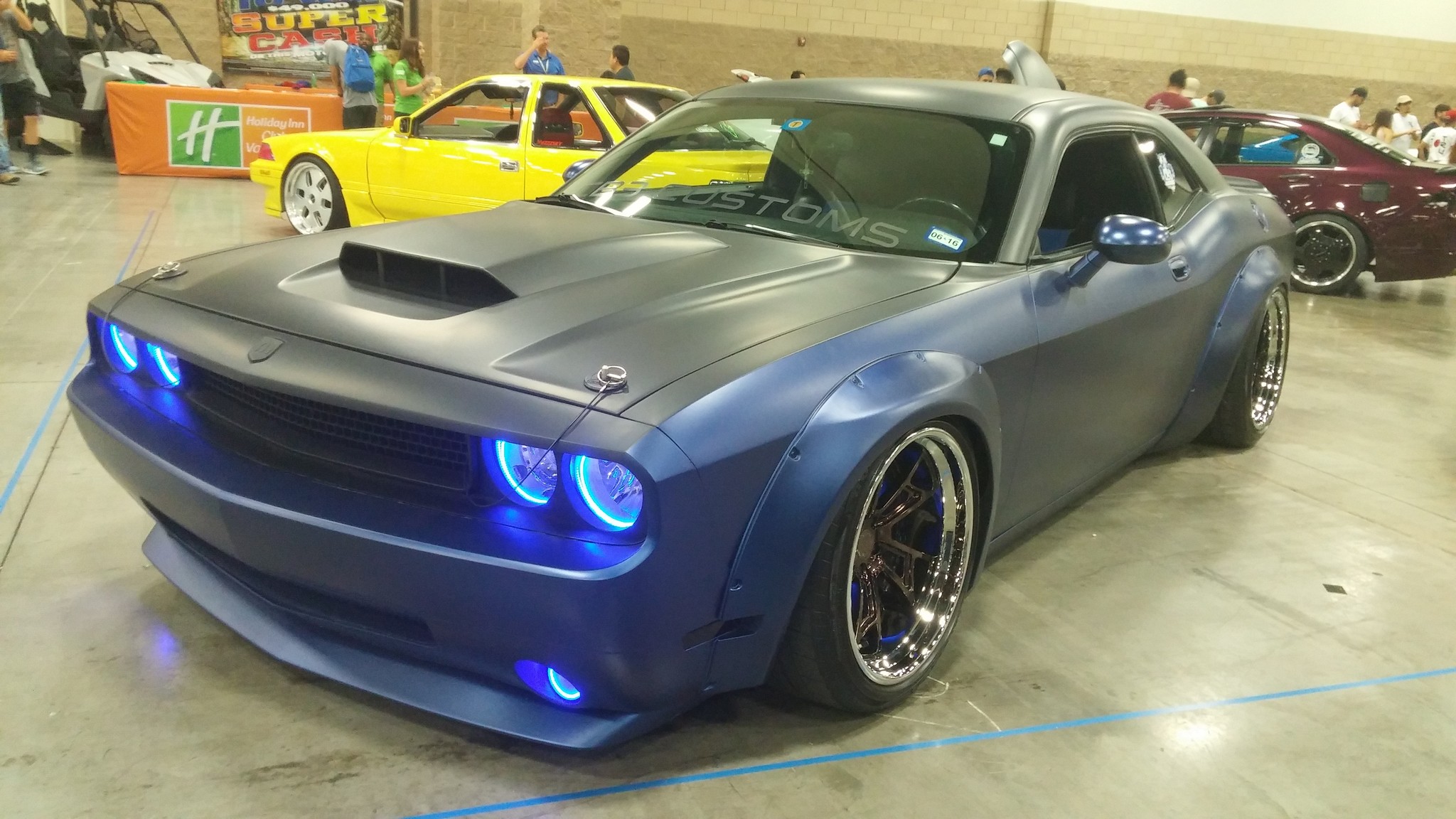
[1144,69,1196,141]
[515,25,565,109]
[791,71,806,79]
[977,68,1014,83]
[1391,95,1422,155]
[357,33,396,128]
[391,37,433,121]
[1366,108,1393,144]
[1191,89,1225,108]
[606,45,635,118]
[324,39,377,129]
[0,0,49,183]
[1417,103,1456,165]
[1331,86,1371,131]
[1181,78,1199,101]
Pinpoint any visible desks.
[105,80,602,179]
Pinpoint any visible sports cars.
[62,76,1301,752]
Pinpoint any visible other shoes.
[7,164,21,174]
[0,174,19,183]
[22,162,47,175]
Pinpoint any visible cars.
[725,68,783,150]
[1155,102,1456,296]
[247,72,774,235]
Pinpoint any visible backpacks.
[337,43,374,93]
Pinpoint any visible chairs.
[538,108,574,146]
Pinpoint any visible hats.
[1397,96,1411,104]
[1212,89,1225,105]
[979,68,994,76]
[1181,78,1200,97]
[1445,110,1456,121]
